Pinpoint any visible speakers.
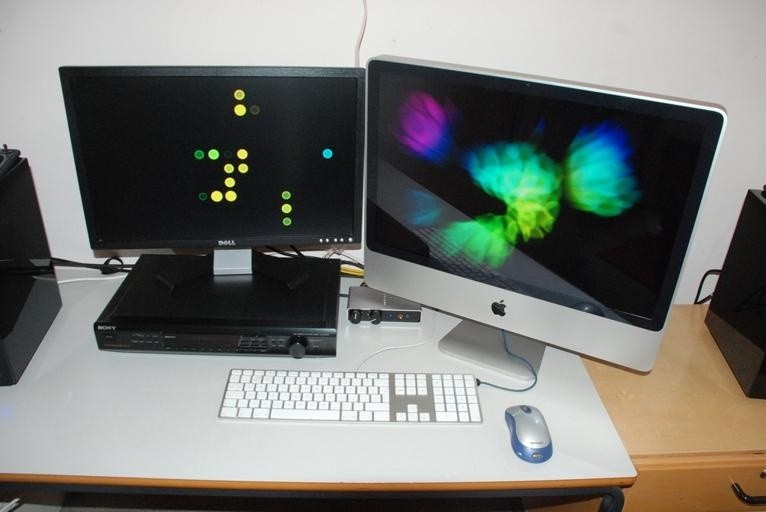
[704,184,766,399]
[0,156,62,387]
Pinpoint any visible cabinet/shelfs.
[518,305,765,512]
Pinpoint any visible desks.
[0,251,636,512]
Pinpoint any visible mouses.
[505,405,554,464]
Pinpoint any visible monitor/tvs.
[59,66,367,276]
[363,54,730,391]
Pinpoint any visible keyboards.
[218,368,483,424]
